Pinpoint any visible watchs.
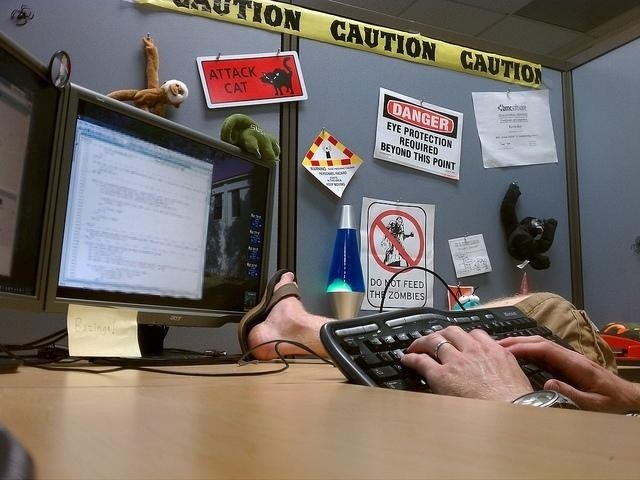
[510,389,580,411]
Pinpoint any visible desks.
[0,358,640,479]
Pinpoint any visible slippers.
[238,269,301,360]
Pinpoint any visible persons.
[235,266,640,416]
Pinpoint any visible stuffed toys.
[500,180,558,270]
[221,113,282,163]
[106,33,189,118]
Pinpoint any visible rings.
[433,339,454,359]
